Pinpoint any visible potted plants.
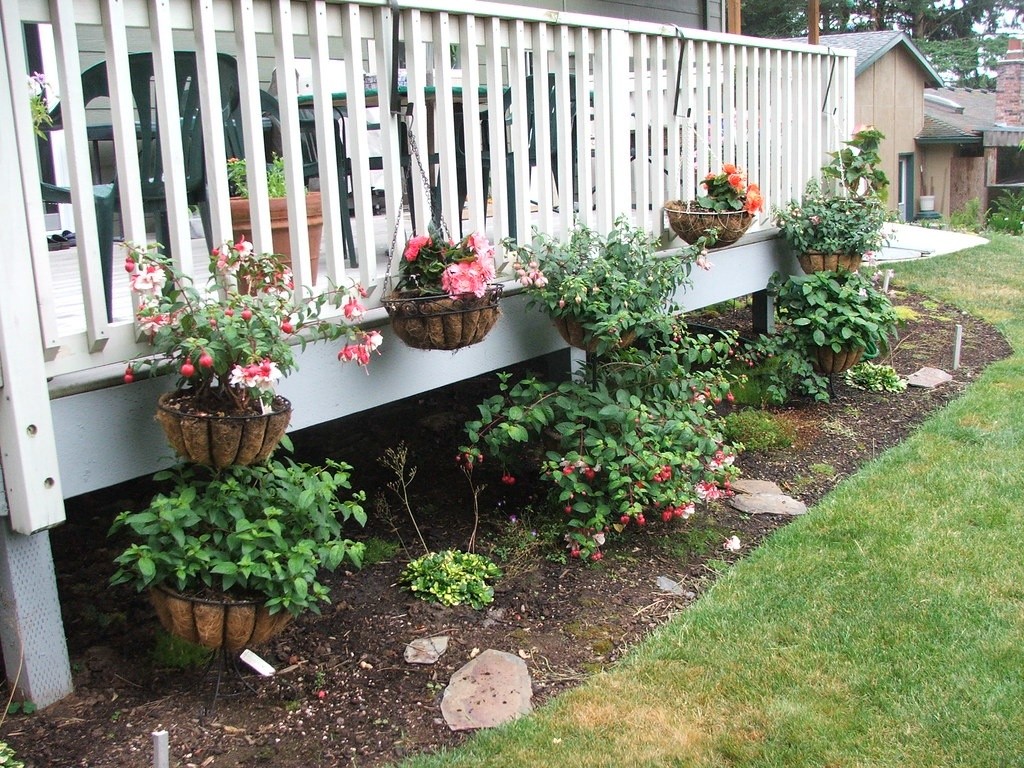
[106,433,367,716]
[764,268,904,405]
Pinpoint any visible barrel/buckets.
[919,195,935,210]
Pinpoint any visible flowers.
[222,152,307,197]
[696,164,763,212]
[497,212,722,357]
[397,219,495,299]
[772,176,885,257]
[118,235,383,415]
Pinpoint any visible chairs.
[35,51,358,324]
[435,73,577,250]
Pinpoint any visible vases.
[548,304,638,353]
[664,201,752,249]
[230,192,323,297]
[379,283,505,350]
[158,387,292,466]
[796,249,862,274]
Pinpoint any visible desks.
[298,85,510,268]
[86,116,280,242]
